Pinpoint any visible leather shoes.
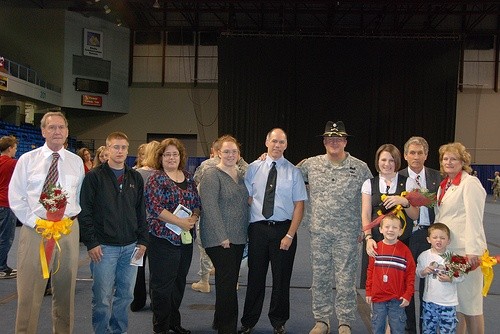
[274,326,287,334]
[238,327,250,334]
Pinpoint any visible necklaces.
[445,178,453,191]
[164,170,179,182]
[380,240,397,283]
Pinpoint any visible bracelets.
[363,233,374,241]
[192,213,200,220]
[285,233,294,239]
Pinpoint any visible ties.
[262,162,277,219]
[39,152,60,203]
[416,175,421,225]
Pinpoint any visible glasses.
[163,152,180,157]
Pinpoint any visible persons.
[361,144,420,334]
[130,138,202,334]
[78,144,109,173]
[488,170,500,202]
[434,141,486,334]
[0,134,18,279]
[79,131,151,334]
[415,222,466,334]
[397,135,443,334]
[191,134,267,334]
[7,112,85,334]
[296,120,375,334]
[365,213,416,334]
[244,127,309,334]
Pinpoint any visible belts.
[257,220,289,226]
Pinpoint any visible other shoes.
[309,321,330,334]
[198,267,215,276]
[338,325,351,334]
[191,280,210,293]
[0,269,17,278]
[170,326,191,334]
[130,304,152,313]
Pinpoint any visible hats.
[319,121,351,137]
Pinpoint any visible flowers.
[40,185,70,275]
[362,188,438,231]
[435,253,500,278]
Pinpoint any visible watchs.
[402,202,411,209]
